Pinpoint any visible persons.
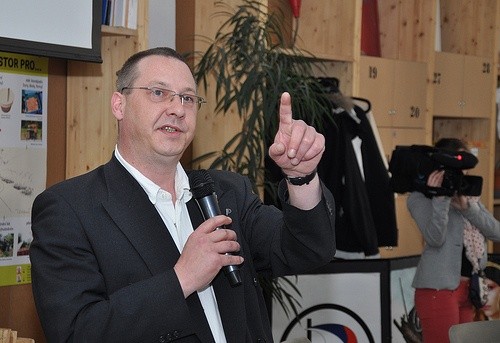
[29,47,337,343]
[470,261,500,321]
[406,138,500,343]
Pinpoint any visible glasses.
[121,85,207,109]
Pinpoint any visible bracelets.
[283,167,317,186]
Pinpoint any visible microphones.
[190,169,243,287]
